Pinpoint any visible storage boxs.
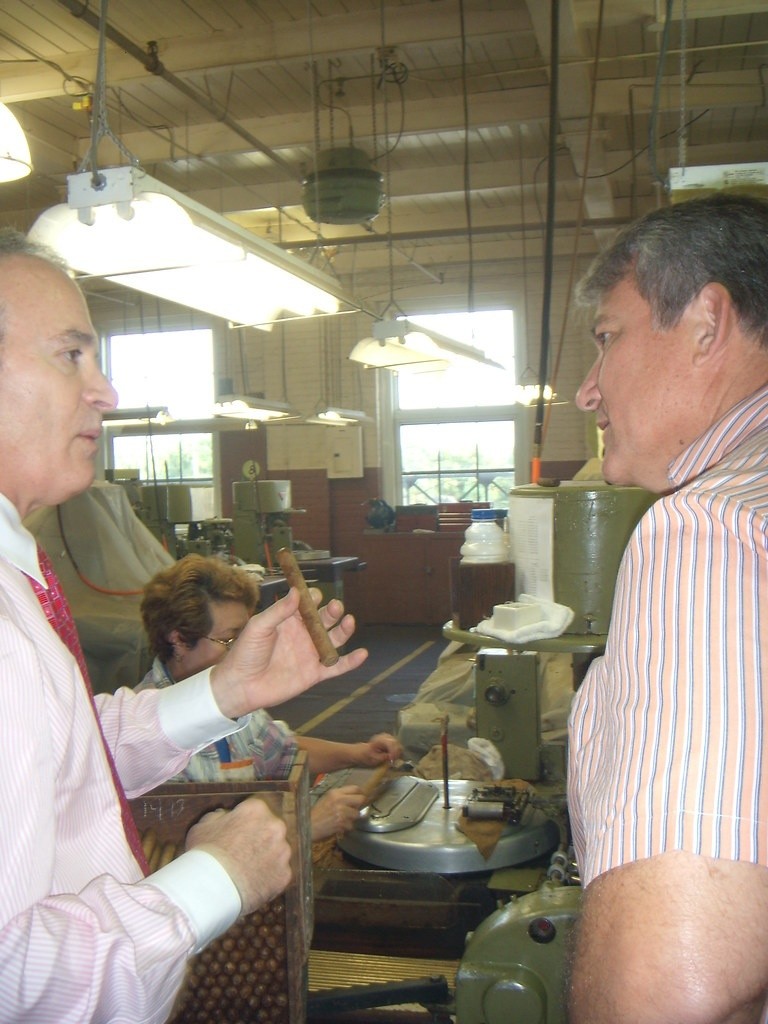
[437,500,494,534]
[124,748,317,1024]
[394,503,439,532]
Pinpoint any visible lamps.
[0,101,558,428]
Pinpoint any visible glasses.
[199,633,238,652]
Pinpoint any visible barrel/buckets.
[457,509,509,564]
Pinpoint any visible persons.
[0,228,372,1024]
[566,189,768,1024]
[130,553,406,846]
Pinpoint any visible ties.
[22,541,152,880]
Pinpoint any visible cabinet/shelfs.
[355,532,464,626]
